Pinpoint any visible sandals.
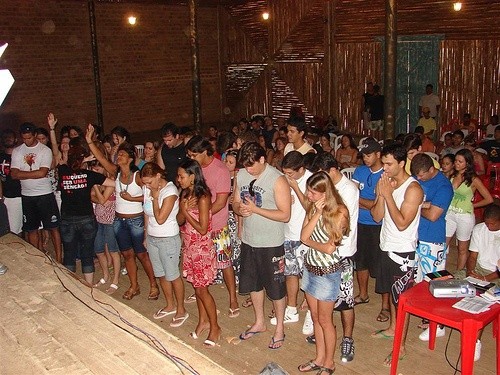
[148,287,160,300]
[242,298,253,308]
[354,295,370,306]
[122,285,140,300]
[376,309,390,322]
[268,310,276,318]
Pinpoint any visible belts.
[304,253,343,276]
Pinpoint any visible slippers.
[153,308,176,319]
[316,367,336,375]
[370,330,394,339]
[184,293,196,303]
[239,324,267,340]
[105,283,120,294]
[228,307,240,317]
[298,359,323,372]
[383,352,407,367]
[170,313,189,327]
[268,334,286,349]
[93,278,112,287]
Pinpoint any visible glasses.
[188,153,200,158]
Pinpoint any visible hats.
[360,142,381,154]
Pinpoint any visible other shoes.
[417,319,429,330]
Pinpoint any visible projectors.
[429,280,476,298]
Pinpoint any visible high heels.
[188,326,210,339]
[202,326,221,349]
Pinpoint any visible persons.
[9,122,62,262]
[231,140,292,350]
[409,153,454,281]
[419,84,441,120]
[176,158,223,349]
[351,135,392,323]
[0,126,24,237]
[207,112,500,318]
[56,146,114,284]
[447,149,494,270]
[34,127,50,252]
[270,149,316,336]
[47,112,70,216]
[283,115,318,157]
[140,162,190,328]
[305,151,356,363]
[90,158,121,295]
[370,142,424,368]
[184,134,242,318]
[361,83,386,133]
[85,123,161,300]
[415,106,437,140]
[61,116,203,275]
[299,171,352,375]
[418,202,500,361]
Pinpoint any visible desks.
[390,280,500,375]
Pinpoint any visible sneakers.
[474,339,481,361]
[340,337,355,362]
[270,305,299,325]
[419,325,445,341]
[302,310,314,334]
[306,335,316,344]
